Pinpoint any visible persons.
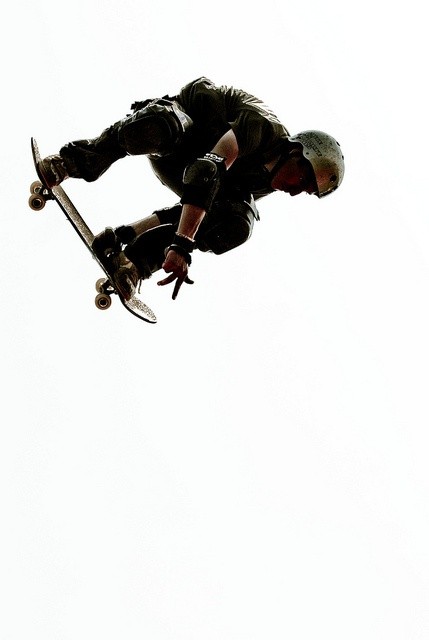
[37,81,348,300]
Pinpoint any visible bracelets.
[173,232,196,253]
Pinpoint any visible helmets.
[287,130,344,199]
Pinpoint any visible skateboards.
[26,136,159,326]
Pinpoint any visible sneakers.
[39,153,69,188]
[112,249,140,301]
